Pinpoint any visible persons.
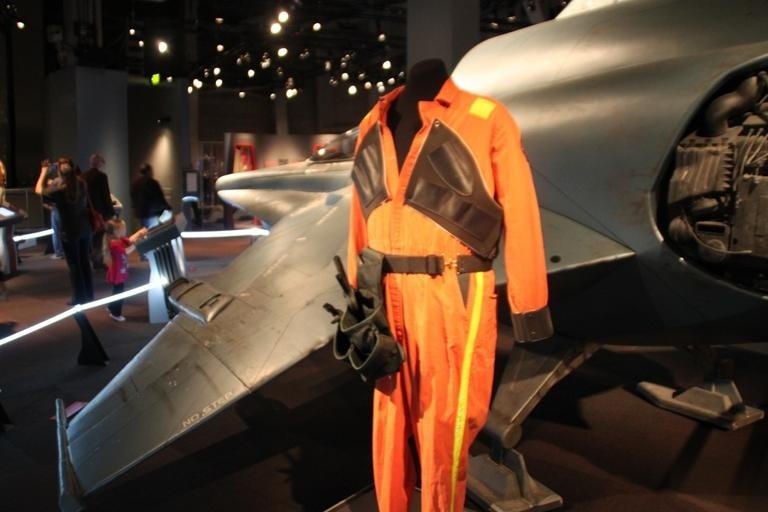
[82,152,114,269]
[131,163,172,264]
[35,158,97,305]
[338,57,558,512]
[41,159,70,261]
[102,216,150,323]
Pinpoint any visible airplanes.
[46,0,768,511]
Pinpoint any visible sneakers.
[106,303,127,322]
